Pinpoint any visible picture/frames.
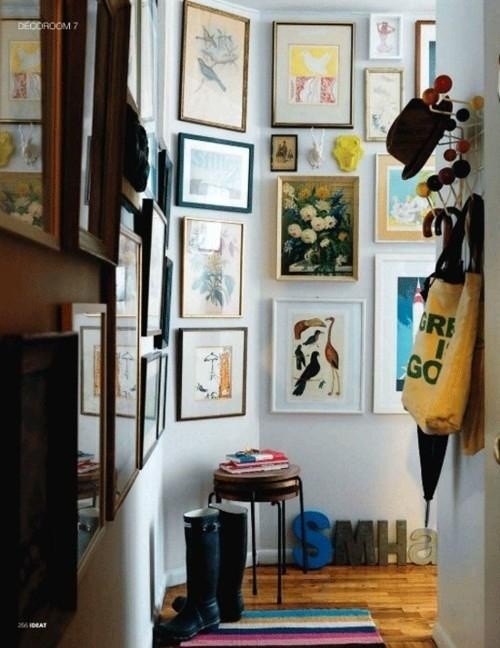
[270,134,298,172]
[0,330,80,648]
[176,132,254,213]
[156,353,168,441]
[116,327,137,419]
[0,15,42,123]
[1,0,63,253]
[415,20,436,99]
[374,153,436,243]
[178,0,251,133]
[269,296,367,415]
[108,222,142,522]
[271,20,356,130]
[80,325,101,416]
[59,301,108,583]
[372,252,437,416]
[275,174,361,283]
[63,0,131,267]
[154,256,173,349]
[141,351,162,471]
[176,327,250,422]
[180,216,246,319]
[158,149,173,257]
[368,13,403,60]
[128,0,141,117]
[364,68,406,142]
[142,197,168,336]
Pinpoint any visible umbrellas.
[416,206,465,527]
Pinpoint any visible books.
[219,449,289,474]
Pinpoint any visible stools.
[208,465,307,605]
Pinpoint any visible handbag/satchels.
[402,195,484,434]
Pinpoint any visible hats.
[386,97,454,181]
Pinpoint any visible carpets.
[178,608,386,648]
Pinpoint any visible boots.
[161,507,222,640]
[173,503,248,622]
